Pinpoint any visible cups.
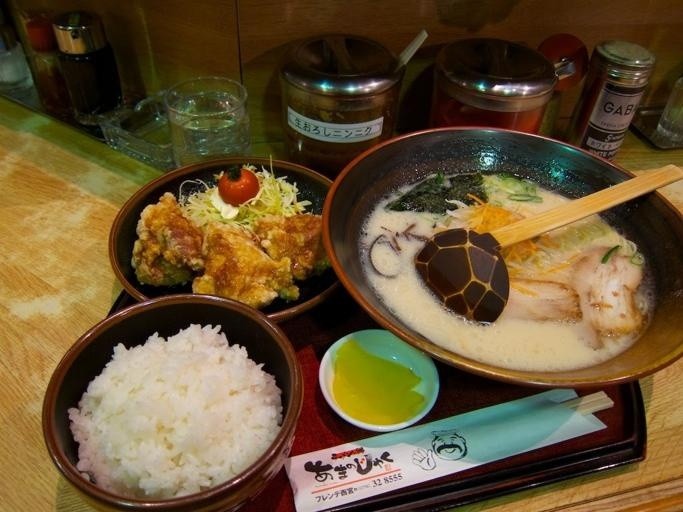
[0,0,128,127]
[165,77,251,168]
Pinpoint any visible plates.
[312,328,440,431]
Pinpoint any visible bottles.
[654,64,683,150]
[427,36,557,136]
[273,31,404,176]
[542,31,590,138]
[583,39,656,160]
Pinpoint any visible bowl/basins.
[111,156,344,336]
[44,293,297,512]
[322,126,682,390]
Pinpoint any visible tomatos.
[219,166,260,206]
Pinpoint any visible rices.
[66,321,284,501]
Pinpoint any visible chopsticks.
[559,391,615,416]
